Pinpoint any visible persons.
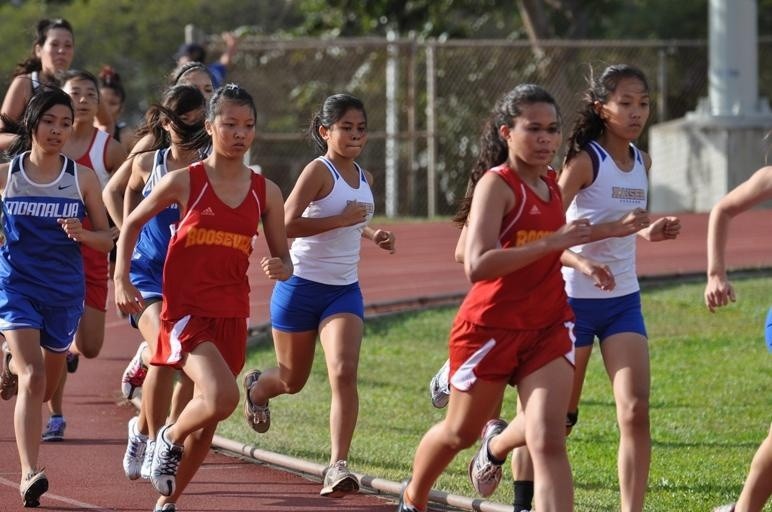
[705,166,772,512]
[241,93,395,501]
[0,16,140,508]
[396,83,613,512]
[98,26,294,511]
[429,164,534,512]
[471,62,682,512]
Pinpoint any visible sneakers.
[711,502,735,512]
[0,342,17,399]
[123,414,184,496]
[399,476,428,511]
[318,457,360,498]
[66,348,79,373]
[242,369,270,433]
[468,419,509,498]
[153,502,178,511]
[19,471,49,507]
[122,340,150,400]
[40,415,67,441]
[429,358,451,408]
